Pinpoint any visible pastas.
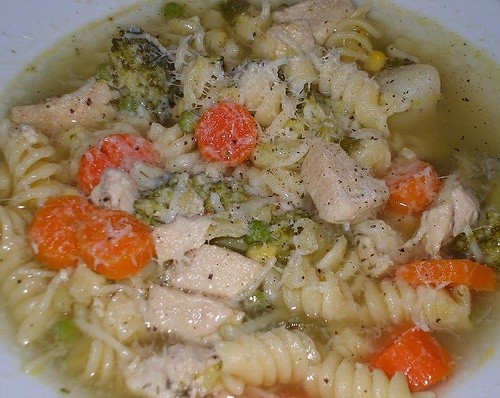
[0,1,500,398]
